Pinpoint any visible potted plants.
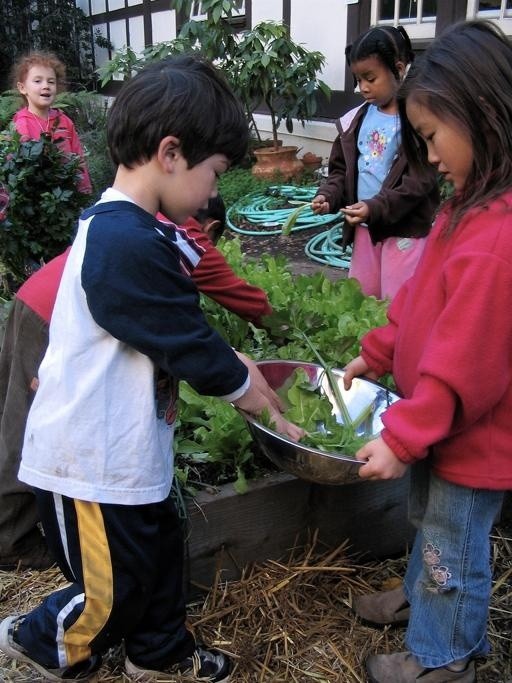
[236,21,334,185]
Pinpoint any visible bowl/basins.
[229,360,413,487]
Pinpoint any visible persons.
[2,52,308,683]
[343,18,512,683]
[1,192,273,570]
[311,26,440,301]
[0,53,92,221]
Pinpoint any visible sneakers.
[125,647,232,683]
[0,614,102,683]
[0,540,58,570]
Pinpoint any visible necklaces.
[30,107,52,140]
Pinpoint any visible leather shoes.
[364,651,476,682]
[353,587,409,629]
